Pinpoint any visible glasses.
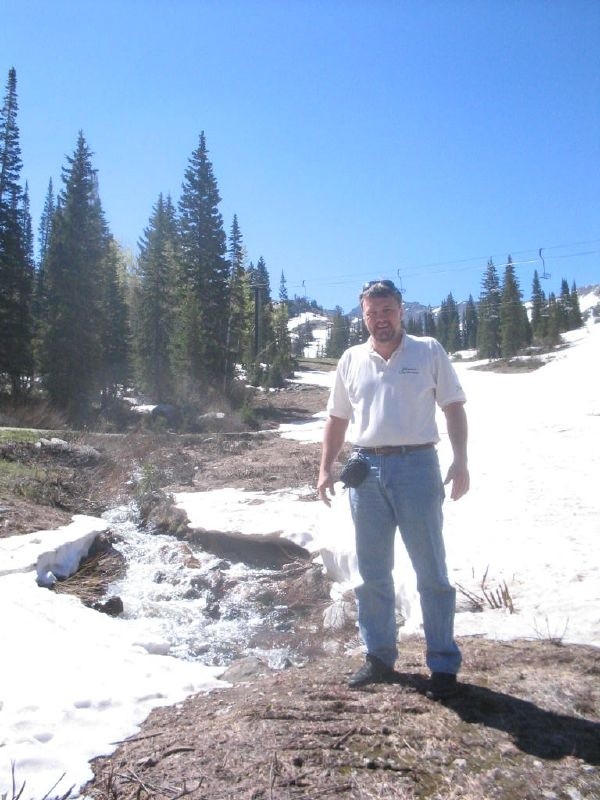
[360,279,398,299]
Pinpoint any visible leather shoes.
[349,653,393,686]
[426,672,457,698]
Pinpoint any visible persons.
[315,278,470,700]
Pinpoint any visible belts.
[355,442,434,455]
